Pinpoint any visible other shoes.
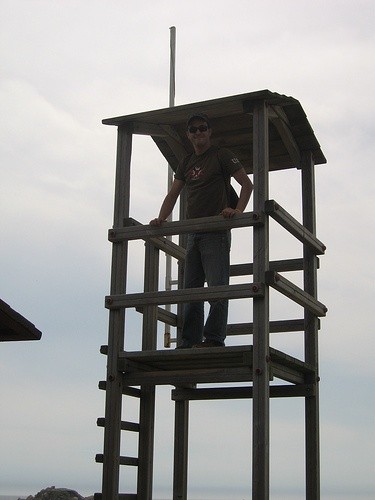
[200,338,224,347]
[175,341,199,347]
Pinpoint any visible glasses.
[188,125,208,133]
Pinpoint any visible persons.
[148,112,255,349]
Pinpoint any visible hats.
[187,113,211,126]
